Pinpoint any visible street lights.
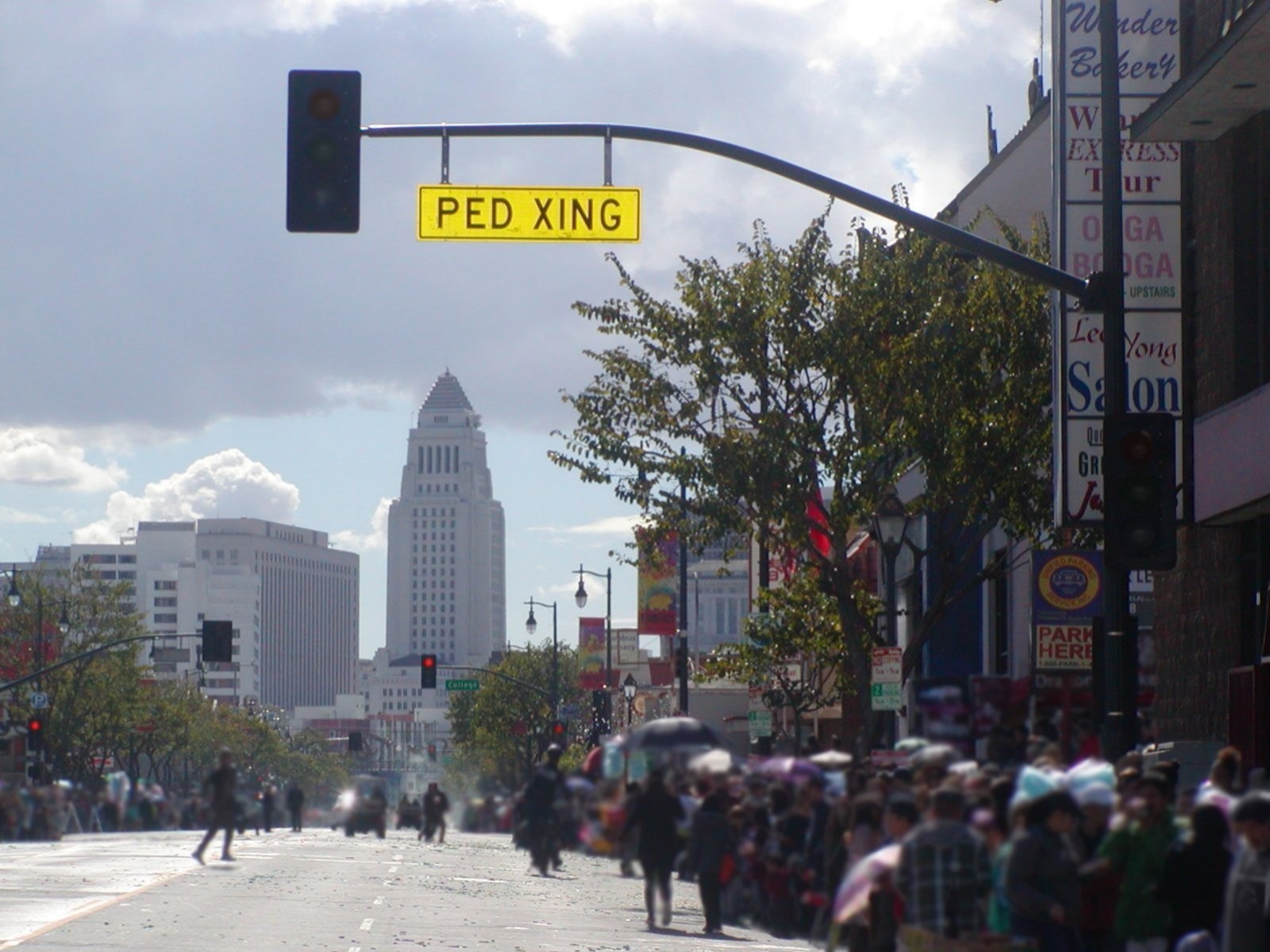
[571,564,614,732]
[875,486,911,751]
[624,673,637,724]
[633,447,690,718]
[184,663,207,797]
[525,596,558,719]
[37,597,69,721]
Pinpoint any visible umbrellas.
[618,716,719,764]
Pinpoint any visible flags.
[578,617,605,689]
[649,660,673,687]
[637,528,677,636]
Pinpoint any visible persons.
[192,746,241,868]
[1,771,451,843]
[463,737,1270,952]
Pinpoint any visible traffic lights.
[1104,410,1180,575]
[428,744,437,762]
[28,717,42,753]
[551,720,568,750]
[419,651,438,688]
[287,68,361,236]
